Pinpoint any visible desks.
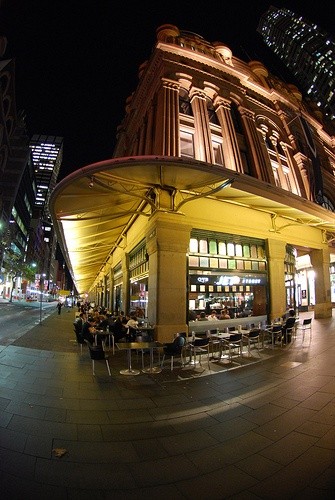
[91,330,113,346]
[116,342,167,375]
[189,315,267,333]
[183,338,202,364]
[230,330,250,353]
[212,333,234,358]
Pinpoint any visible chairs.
[73,312,311,376]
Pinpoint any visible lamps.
[156,184,176,196]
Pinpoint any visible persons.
[82,318,95,344]
[208,310,217,319]
[125,316,138,335]
[136,308,144,318]
[199,312,208,321]
[75,302,127,346]
[219,310,230,319]
[122,315,130,324]
[224,307,231,315]
[57,302,62,314]
[288,305,294,310]
[77,301,80,312]
